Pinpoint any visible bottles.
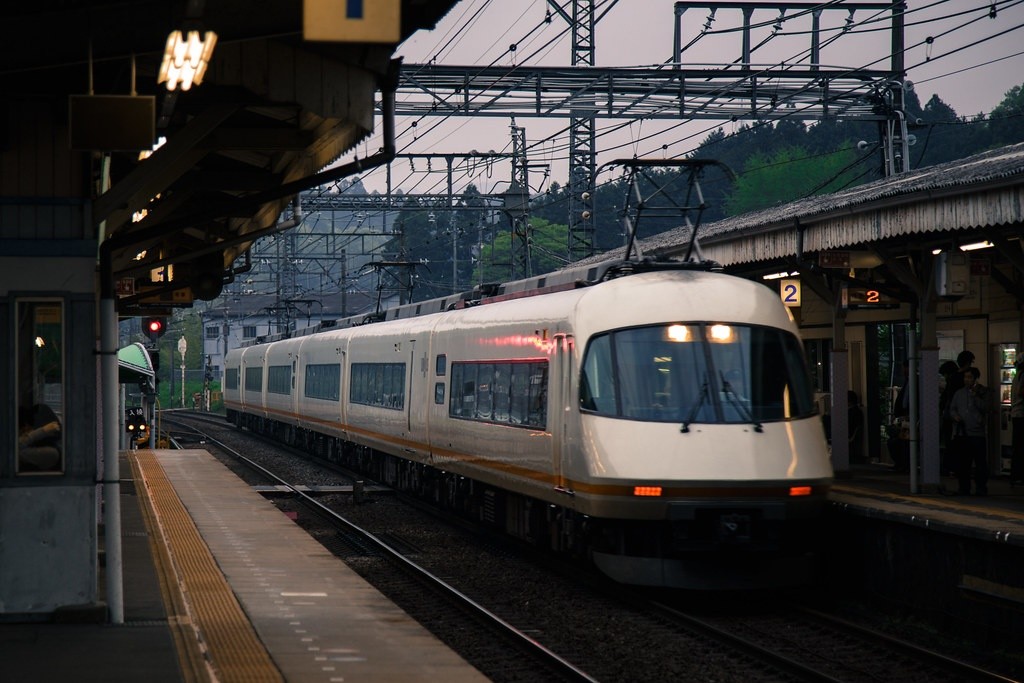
[982,392,991,400]
[1002,413,1007,430]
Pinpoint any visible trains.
[223,158,834,571]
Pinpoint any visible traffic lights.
[142,316,167,340]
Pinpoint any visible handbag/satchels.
[899,418,911,439]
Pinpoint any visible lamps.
[157,18,218,90]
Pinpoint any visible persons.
[720,368,751,402]
[18,404,61,471]
[654,371,671,406]
[457,380,489,418]
[1009,351,1024,483]
[956,351,975,389]
[950,366,993,498]
[887,356,922,485]
[939,360,965,478]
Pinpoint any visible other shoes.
[954,489,971,495]
[976,488,988,495]
